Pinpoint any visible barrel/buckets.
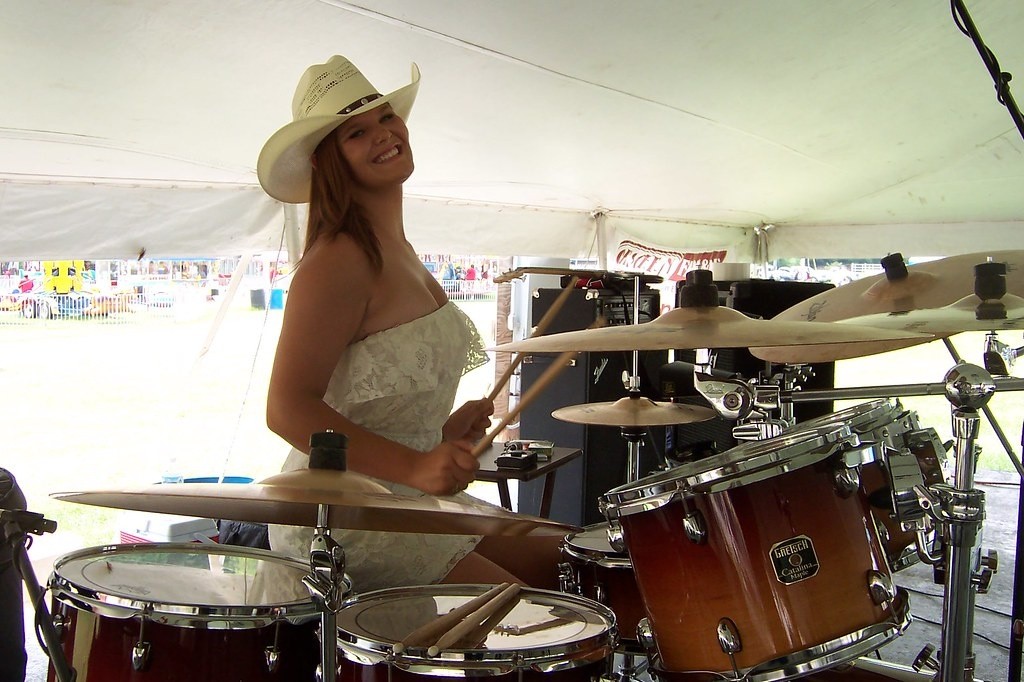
[250,289,265,310]
[269,289,283,309]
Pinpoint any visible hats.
[256,56,423,205]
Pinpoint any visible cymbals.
[480,304,936,353]
[550,394,717,426]
[747,248,1024,365]
[48,463,587,537]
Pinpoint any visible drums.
[784,395,951,573]
[555,515,656,656]
[45,542,355,682]
[328,579,619,682]
[596,419,915,682]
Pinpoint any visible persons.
[794,267,811,281]
[257,55,577,593]
[18,275,34,294]
[270,267,283,285]
[5,269,10,285]
[452,265,490,294]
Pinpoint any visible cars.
[420,261,460,291]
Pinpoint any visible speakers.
[705,280,835,430]
[518,349,670,528]
